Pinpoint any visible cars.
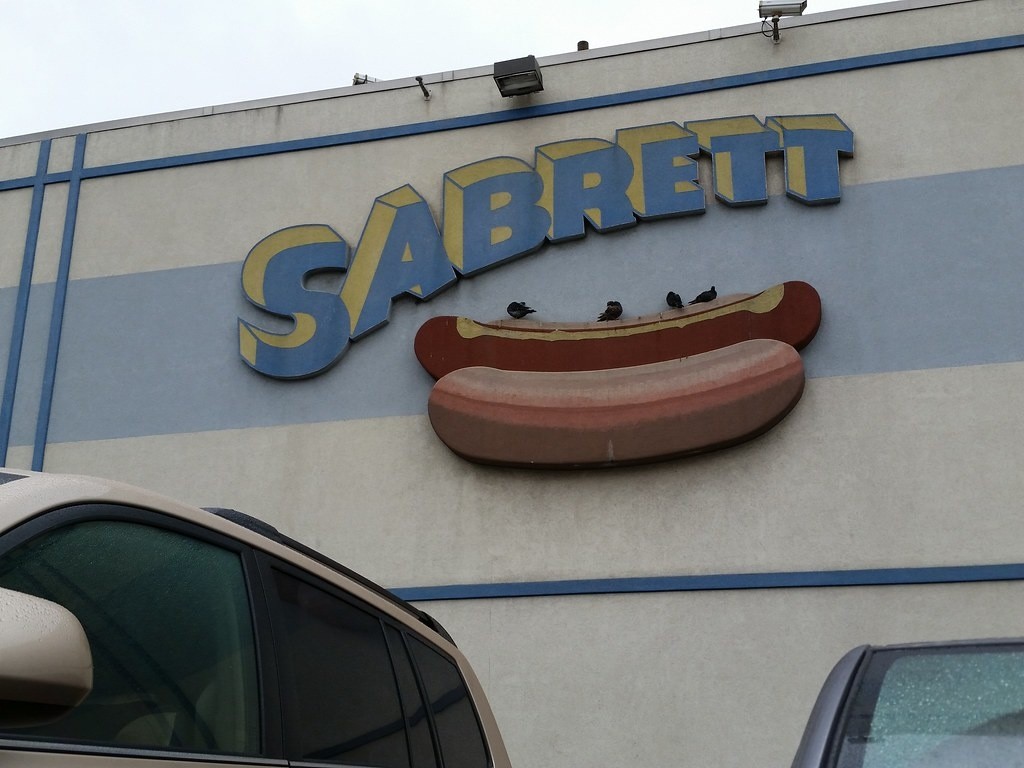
[791,635,1024,768]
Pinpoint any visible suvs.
[0,468,514,768]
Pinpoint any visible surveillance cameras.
[759,0,808,18]
